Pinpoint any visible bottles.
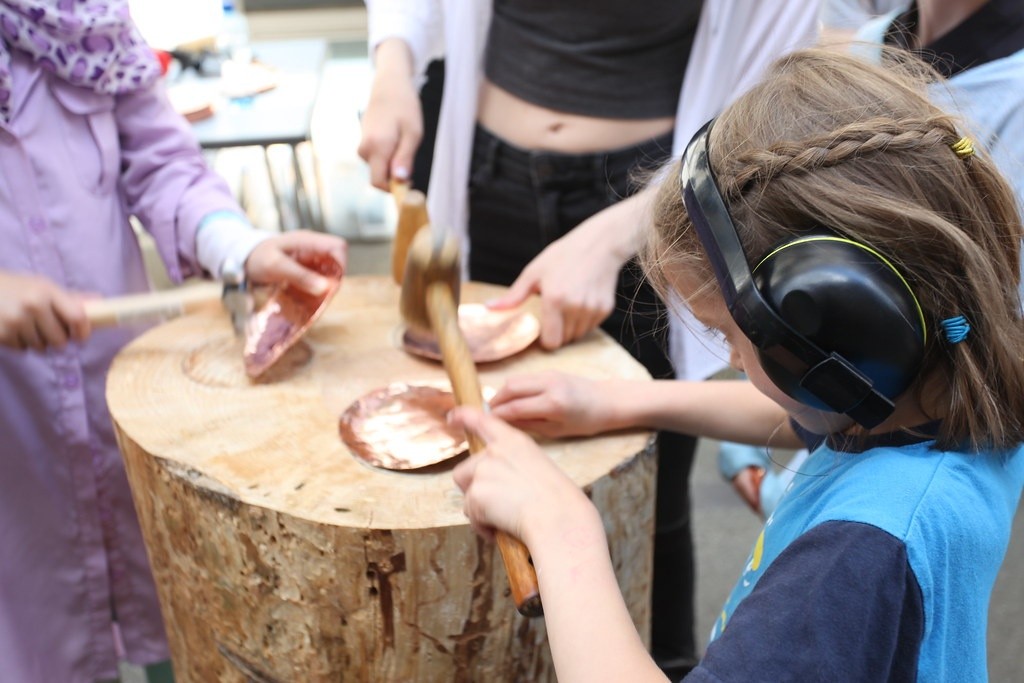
[217,0,253,106]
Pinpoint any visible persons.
[447,42,1024,682]
[359,1,1024,681]
[0,2,347,683]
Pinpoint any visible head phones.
[673,117,929,433]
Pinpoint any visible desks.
[158,35,330,235]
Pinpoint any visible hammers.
[388,174,430,289]
[83,253,255,339]
[399,221,549,620]
